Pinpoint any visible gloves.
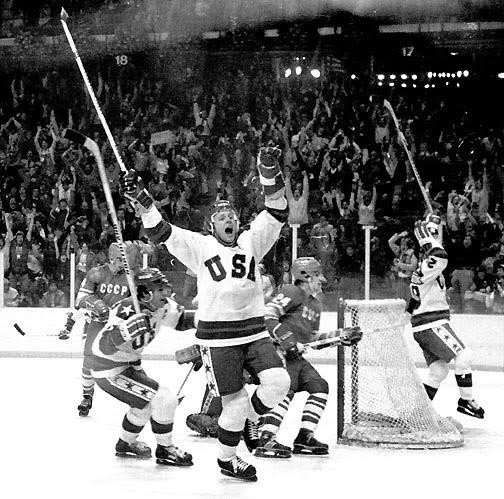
[285,343,305,357]
[328,326,363,348]
[117,168,154,213]
[257,146,282,186]
[413,214,442,251]
[59,329,70,340]
[94,299,108,319]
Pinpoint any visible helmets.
[291,256,328,283]
[109,243,126,264]
[135,265,165,286]
[203,200,241,235]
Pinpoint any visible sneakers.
[458,398,484,418]
[116,438,150,453]
[78,398,91,410]
[155,443,192,463]
[186,415,328,479]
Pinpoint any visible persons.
[256,257,363,453]
[186,255,277,437]
[58,267,99,338]
[78,242,134,413]
[1,52,504,306]
[89,268,195,462]
[120,141,291,480]
[406,214,485,419]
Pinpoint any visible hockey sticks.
[303,296,419,349]
[13,322,86,338]
[109,330,178,361]
[59,6,128,177]
[62,131,140,316]
[368,95,436,214]
